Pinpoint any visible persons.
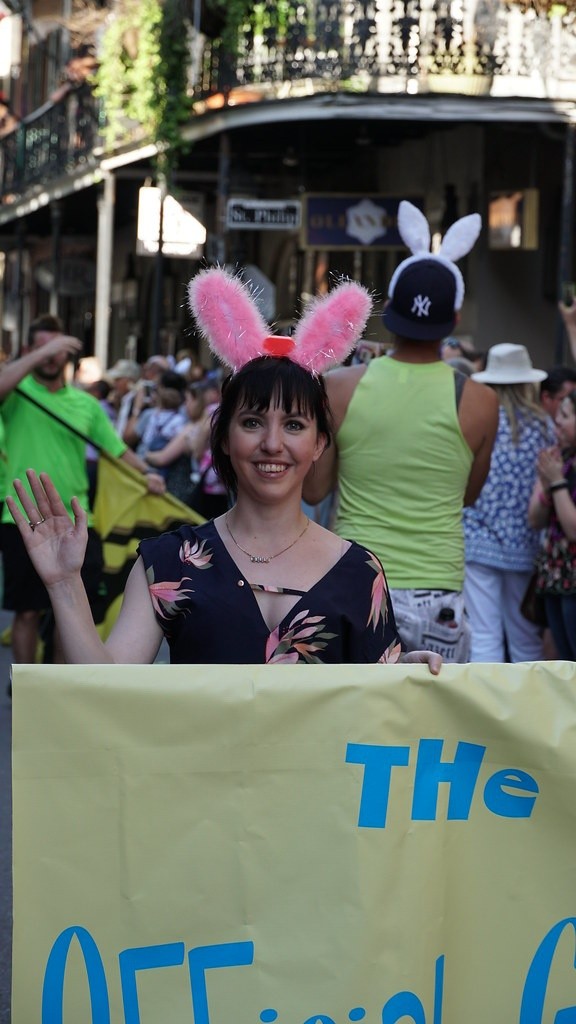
[461,343,558,662]
[0,103,26,191]
[67,42,101,148]
[302,260,499,663]
[0,316,233,698]
[443,337,488,371]
[521,389,576,662]
[539,288,576,417]
[6,355,443,676]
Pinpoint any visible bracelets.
[550,479,569,491]
[144,468,161,473]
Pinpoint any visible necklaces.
[226,508,308,563]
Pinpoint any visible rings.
[30,519,44,526]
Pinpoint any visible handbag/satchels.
[519,572,547,627]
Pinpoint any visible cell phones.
[142,381,154,403]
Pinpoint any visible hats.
[469,343,549,384]
[382,260,456,342]
